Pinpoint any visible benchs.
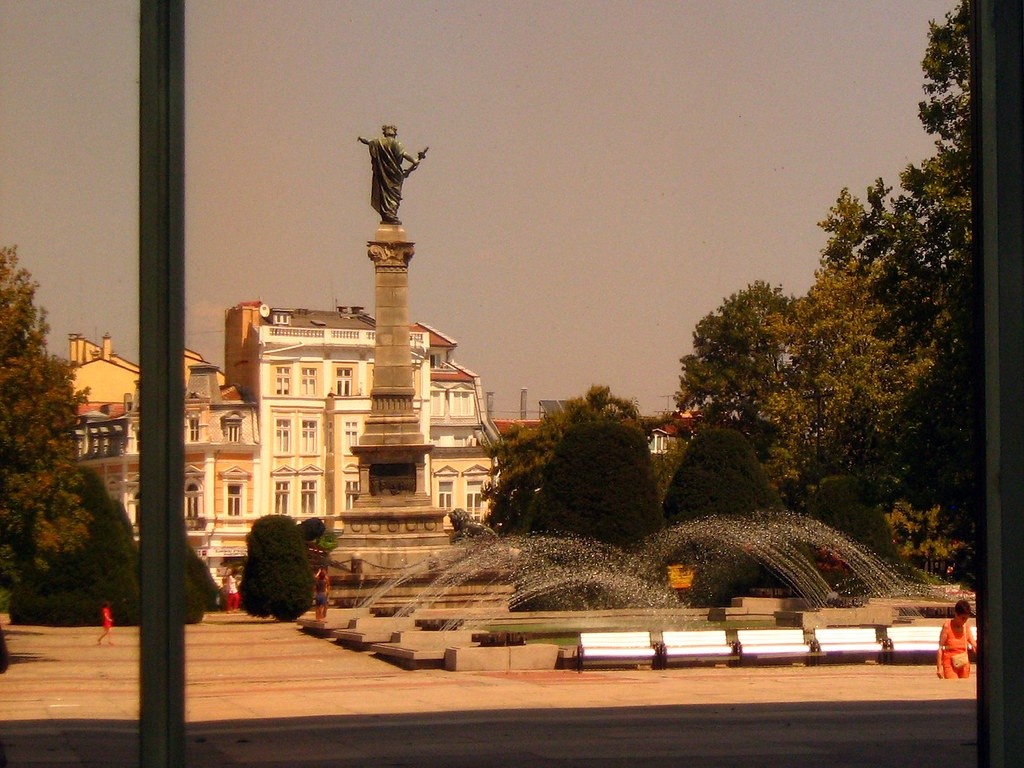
[886,626,978,665]
[737,630,815,667]
[577,631,660,672]
[813,628,886,666]
[661,631,739,670]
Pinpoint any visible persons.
[357,124,421,222]
[98,601,114,645]
[227,568,239,614]
[936,600,977,679]
[315,568,329,621]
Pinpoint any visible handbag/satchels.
[952,652,969,668]
